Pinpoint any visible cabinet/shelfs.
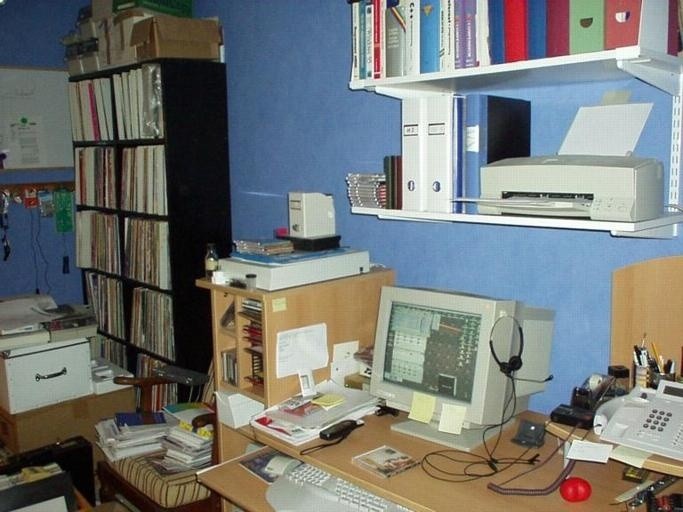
[348,45,683,239]
[68,57,232,411]
[0,338,94,415]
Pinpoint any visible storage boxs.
[130,15,223,60]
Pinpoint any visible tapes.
[589,374,602,391]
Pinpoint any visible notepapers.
[310,394,344,406]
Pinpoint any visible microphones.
[505,374,553,384]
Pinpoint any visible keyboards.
[265,462,415,512]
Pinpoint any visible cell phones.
[319,419,357,441]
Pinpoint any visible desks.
[195,256,683,512]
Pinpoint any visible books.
[135,353,177,412]
[68,64,163,142]
[95,401,215,469]
[84,270,125,340]
[96,333,127,370]
[348,1,491,81]
[75,146,116,209]
[121,145,168,216]
[123,216,172,289]
[252,375,385,447]
[131,287,175,361]
[200,355,215,404]
[75,209,121,276]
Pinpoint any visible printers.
[477,151,665,222]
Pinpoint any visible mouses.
[266,455,294,477]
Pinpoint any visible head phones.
[489,314,524,373]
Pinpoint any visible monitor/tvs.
[369,284,555,453]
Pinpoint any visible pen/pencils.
[633,333,675,374]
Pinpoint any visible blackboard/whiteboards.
[0,66,74,171]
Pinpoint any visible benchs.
[97,359,221,511]
